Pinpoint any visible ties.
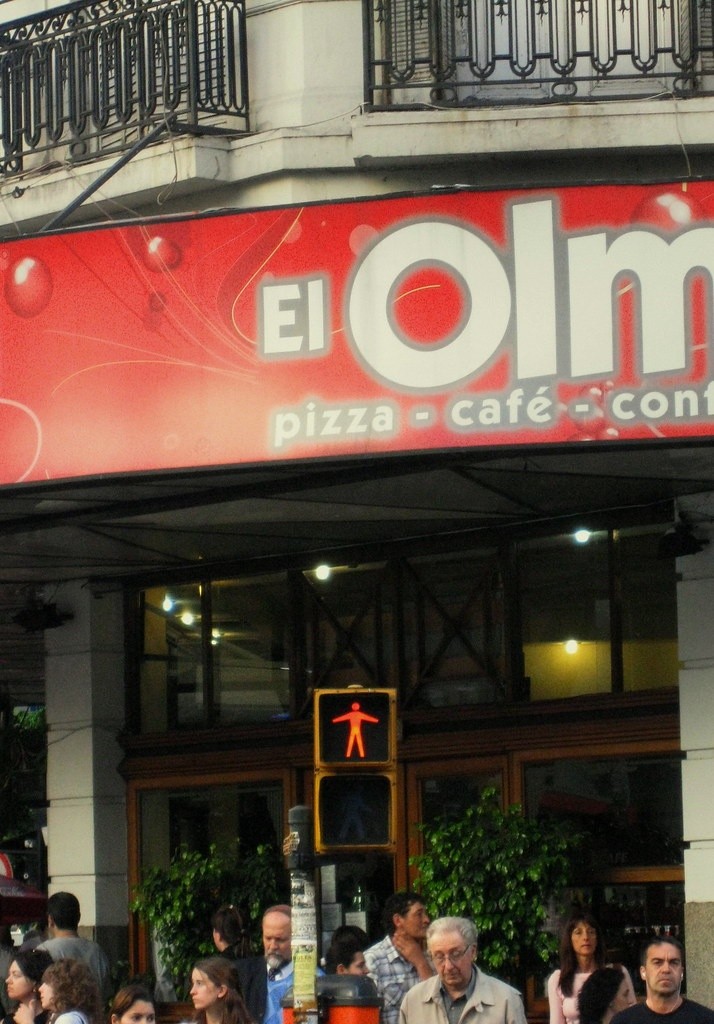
[268,968,281,982]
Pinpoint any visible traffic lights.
[312,686,399,854]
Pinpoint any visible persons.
[0,891,714,1024]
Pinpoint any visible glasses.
[431,945,471,966]
